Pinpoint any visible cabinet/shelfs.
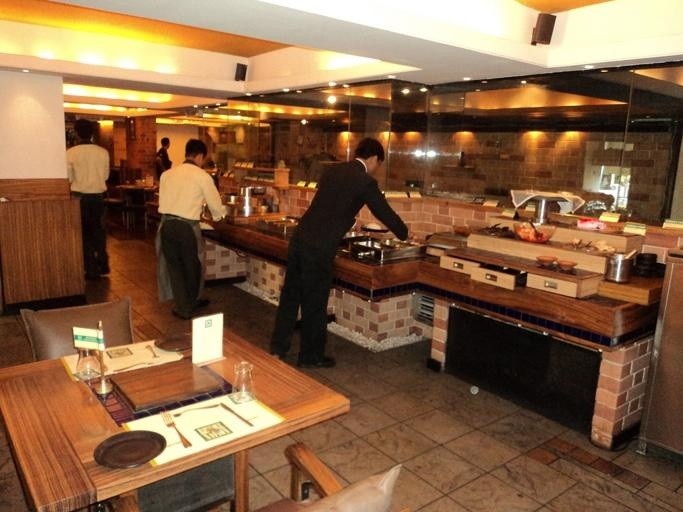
[635,253,683,457]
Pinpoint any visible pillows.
[19,295,138,362]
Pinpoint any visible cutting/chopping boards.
[110,359,220,411]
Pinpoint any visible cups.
[534,198,548,219]
[145,176,154,186]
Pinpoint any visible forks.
[160,411,192,448]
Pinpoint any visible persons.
[271,137,414,368]
[157,138,232,320]
[66,117,111,278]
[156,137,171,181]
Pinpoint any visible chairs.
[249,441,403,512]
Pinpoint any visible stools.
[102,197,127,227]
[125,202,151,230]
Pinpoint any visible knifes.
[220,402,253,427]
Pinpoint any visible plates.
[155,332,191,351]
[94,430,166,468]
[514,223,556,242]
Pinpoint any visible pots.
[605,249,638,283]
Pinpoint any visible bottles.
[233,362,255,403]
[77,348,101,379]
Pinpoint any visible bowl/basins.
[224,192,239,202]
[558,260,577,271]
[537,256,557,266]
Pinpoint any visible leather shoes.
[193,299,208,306]
[271,352,288,359]
[173,309,194,319]
[298,354,336,369]
